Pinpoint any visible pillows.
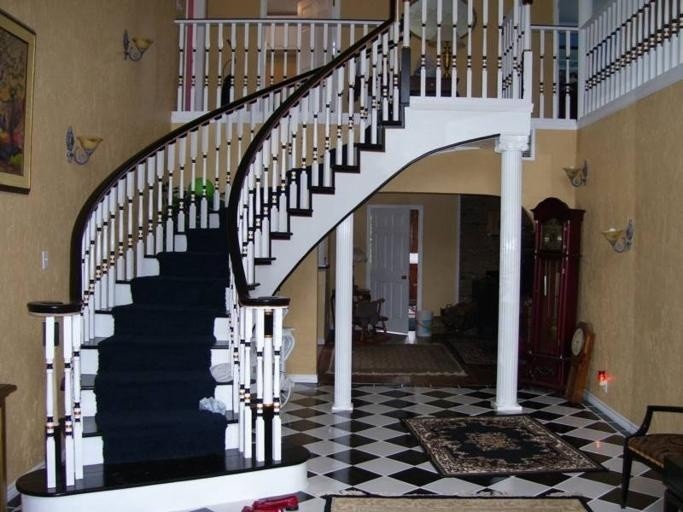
[353,299,376,318]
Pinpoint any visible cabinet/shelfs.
[349,71,460,100]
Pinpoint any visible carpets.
[398,413,609,477]
[324,341,469,378]
[321,493,594,511]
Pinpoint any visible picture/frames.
[0,7,39,196]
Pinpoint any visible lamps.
[560,159,589,188]
[428,22,468,74]
[63,122,102,166]
[352,245,368,288]
[597,368,608,393]
[600,213,632,254]
[122,26,154,64]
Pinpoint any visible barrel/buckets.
[415,309,434,338]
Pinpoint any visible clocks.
[564,317,594,405]
[525,193,585,387]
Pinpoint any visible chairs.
[329,283,389,345]
[619,404,682,512]
